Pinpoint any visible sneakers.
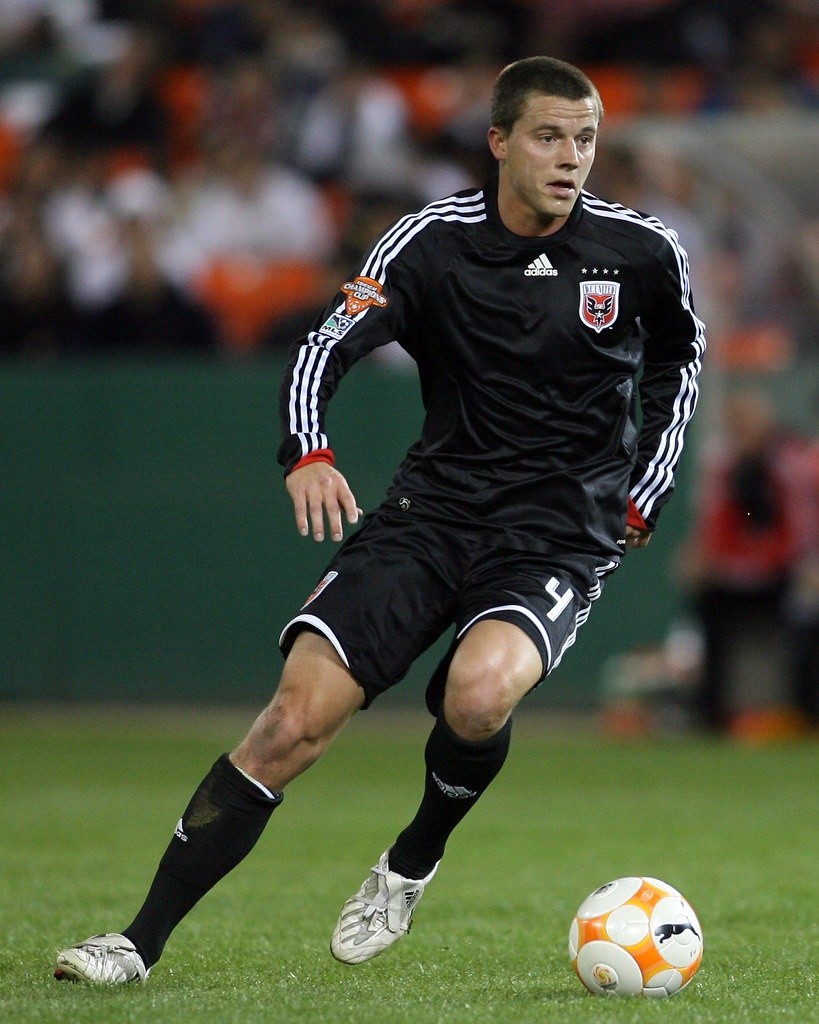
[54,933,162,989]
[330,841,441,966]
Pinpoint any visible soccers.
[568,874,706,999]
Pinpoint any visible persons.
[0,0,819,746]
[55,58,709,987]
